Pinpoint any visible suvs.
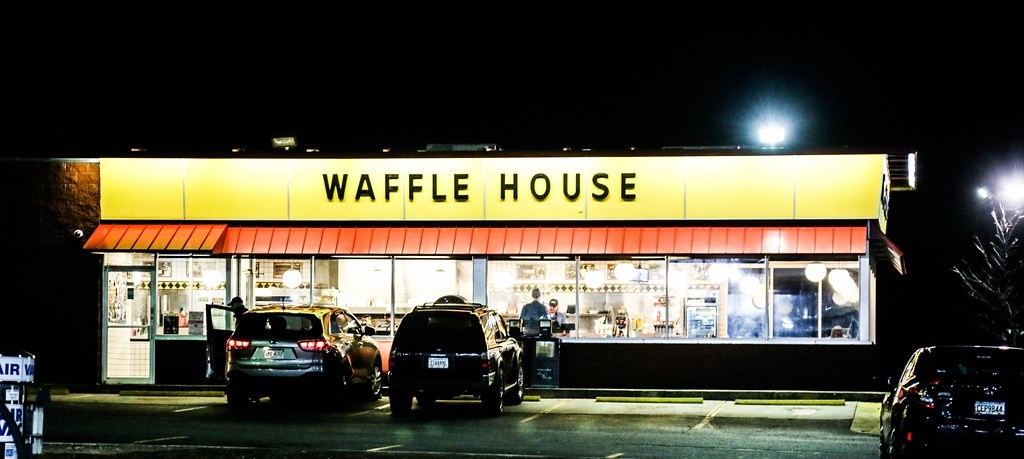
[388,292,526,418]
[205,301,383,420]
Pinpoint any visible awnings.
[211,225,870,259]
[874,230,909,276]
[81,223,226,253]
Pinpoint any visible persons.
[228,296,248,324]
[521,287,549,341]
[540,299,571,341]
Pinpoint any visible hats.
[549,299,558,306]
[228,296,243,305]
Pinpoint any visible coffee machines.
[613,306,629,338]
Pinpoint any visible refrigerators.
[684,278,728,339]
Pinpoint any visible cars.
[872,343,1024,459]
